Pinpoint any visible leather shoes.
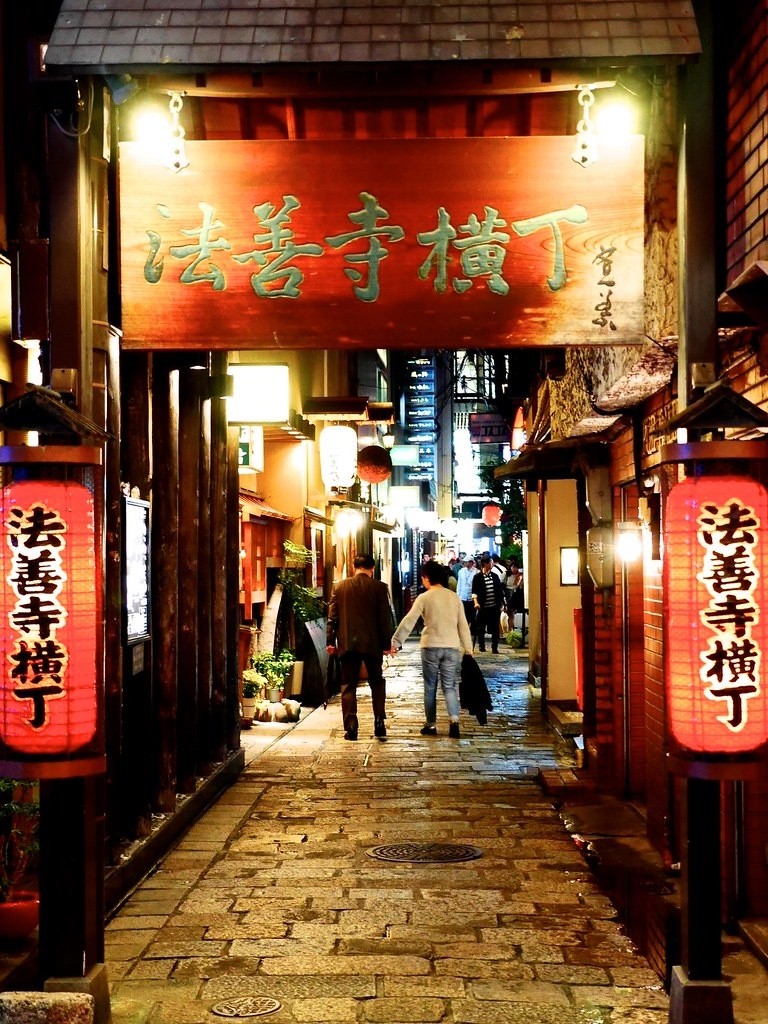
[344,733,358,741]
[375,722,386,736]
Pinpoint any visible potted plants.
[505,628,522,649]
[260,649,296,702]
[252,658,290,703]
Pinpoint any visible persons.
[420,553,431,567]
[390,561,474,739]
[325,552,394,740]
[440,549,523,654]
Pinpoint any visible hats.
[463,555,474,561]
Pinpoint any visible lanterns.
[1,462,100,758]
[481,505,501,528]
[319,425,357,494]
[661,458,768,754]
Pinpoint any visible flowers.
[242,670,269,698]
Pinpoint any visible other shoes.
[420,727,436,735]
[449,723,460,738]
[479,648,486,652]
[492,649,499,653]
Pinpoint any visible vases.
[240,698,256,719]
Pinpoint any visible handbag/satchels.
[500,611,509,634]
[323,653,342,701]
[511,588,527,613]
